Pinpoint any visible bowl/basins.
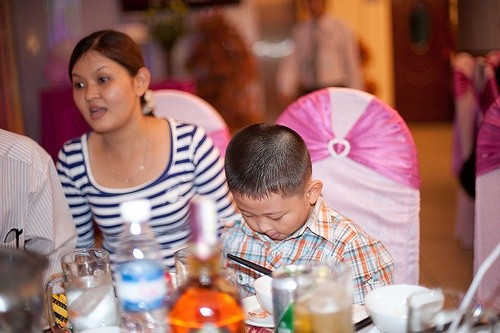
[254,275,274,315]
[364,284,431,333]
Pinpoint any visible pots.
[0,242,51,333]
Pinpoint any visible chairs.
[277,86,420,285]
[150,89,231,245]
[473,95,500,303]
[451,51,484,250]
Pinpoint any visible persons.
[0,127,75,333]
[58,30,237,280]
[217,122,395,306]
[272,0,366,109]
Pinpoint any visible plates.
[242,295,274,329]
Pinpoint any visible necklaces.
[106,119,150,185]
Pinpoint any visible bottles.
[166,195,247,333]
[110,198,174,333]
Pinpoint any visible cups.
[292,258,355,333]
[44,247,120,333]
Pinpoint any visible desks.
[36,78,200,168]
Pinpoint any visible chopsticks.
[227,253,273,277]
